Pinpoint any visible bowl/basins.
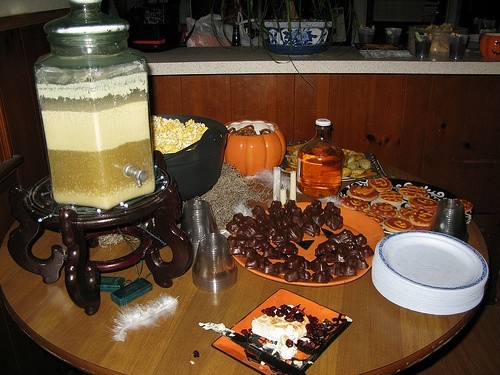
[407,24,479,54]
[151,113,286,201]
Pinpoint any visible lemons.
[414,32,423,42]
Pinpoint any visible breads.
[285,143,378,179]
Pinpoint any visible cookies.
[340,178,472,233]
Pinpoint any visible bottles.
[33,0,155,210]
[232,23,241,47]
[296,118,344,199]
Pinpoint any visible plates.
[232,201,385,286]
[372,231,489,316]
[338,178,472,236]
[281,140,381,182]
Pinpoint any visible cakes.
[251,304,310,359]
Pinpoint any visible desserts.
[226,200,375,284]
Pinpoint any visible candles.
[290,168,297,202]
[273,165,280,202]
[280,184,287,206]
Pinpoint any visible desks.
[0,164,489,375]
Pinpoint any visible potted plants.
[211,0,357,90]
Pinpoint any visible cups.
[414,32,468,60]
[384,27,402,45]
[181,201,218,263]
[192,233,237,291]
[478,18,500,61]
[430,198,469,244]
[358,27,375,45]
